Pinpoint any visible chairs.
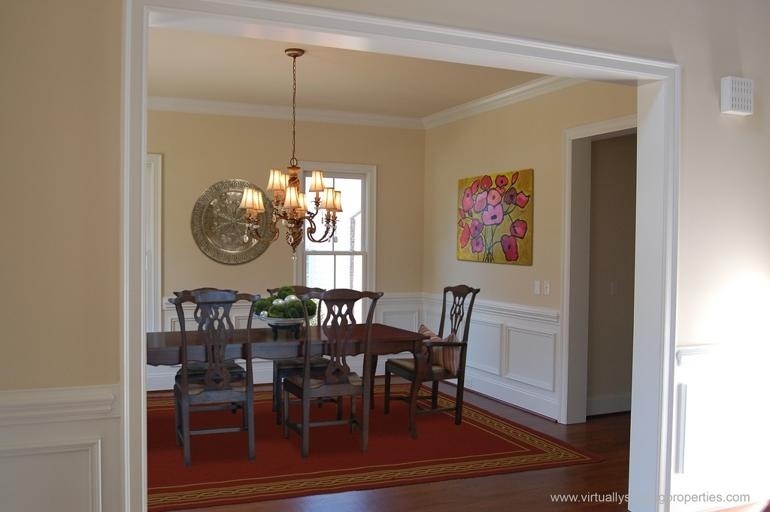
[172,286,240,414]
[167,289,262,470]
[381,281,481,438]
[266,285,343,424]
[279,285,385,460]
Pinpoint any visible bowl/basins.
[252,311,316,327]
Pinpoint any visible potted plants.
[251,284,317,337]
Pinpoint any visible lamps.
[237,45,344,256]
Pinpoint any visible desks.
[147,319,434,439]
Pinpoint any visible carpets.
[146,380,608,511]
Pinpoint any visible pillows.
[416,322,460,376]
